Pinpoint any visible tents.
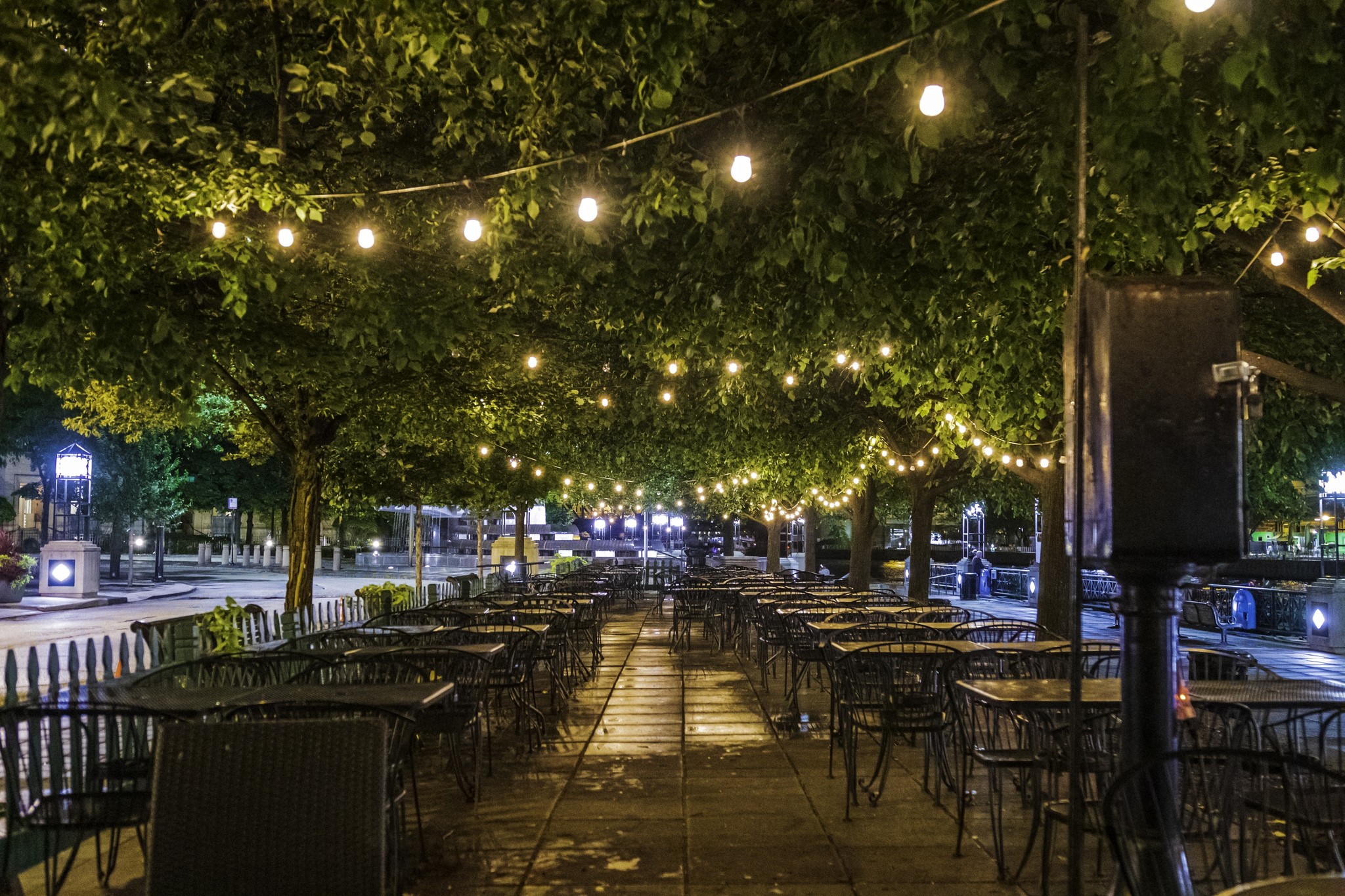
[1266,530,1298,553]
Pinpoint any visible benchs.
[1100,587,1244,648]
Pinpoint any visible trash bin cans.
[1017,547,1021,551]
[979,569,990,592]
[1283,555,1285,560]
[1232,589,1256,629]
[960,572,978,601]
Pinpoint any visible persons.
[972,550,989,596]
[819,564,830,575]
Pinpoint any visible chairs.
[0,561,645,896]
[651,563,1345,896]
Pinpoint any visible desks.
[537,592,608,623]
[729,585,787,637]
[342,642,507,797]
[483,608,577,695]
[505,578,555,593]
[459,600,519,612]
[755,596,834,686]
[784,585,855,593]
[767,578,825,587]
[666,587,732,643]
[325,624,445,648]
[601,571,639,611]
[0,683,264,896]
[479,593,539,602]
[689,581,769,589]
[1180,678,1345,896]
[775,607,873,698]
[202,646,366,691]
[916,621,1048,645]
[803,590,883,597]
[508,608,576,678]
[864,604,965,626]
[975,639,1122,681]
[221,680,462,869]
[434,624,551,753]
[826,639,990,807]
[807,620,924,692]
[556,580,605,592]
[952,673,1205,885]
[422,607,490,627]
[835,596,917,608]
[739,590,806,662]
[523,599,600,665]
